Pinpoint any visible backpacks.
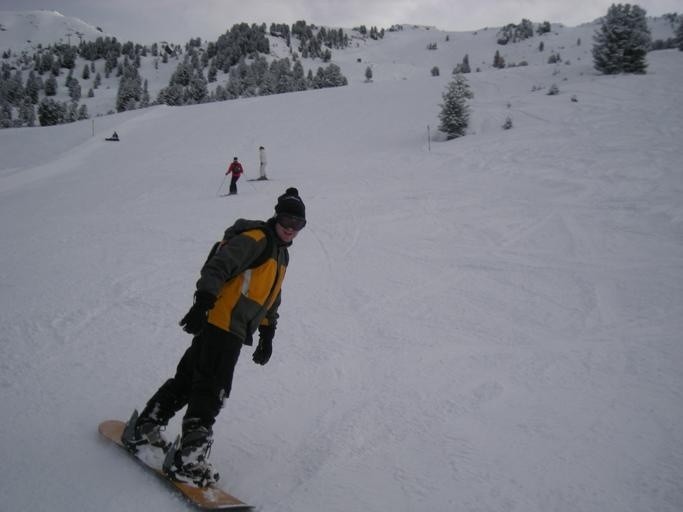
[197,219,289,290]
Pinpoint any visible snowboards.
[98,420,253,508]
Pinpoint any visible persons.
[119,186,310,489]
[255,145,269,181]
[223,155,245,196]
[111,129,119,139]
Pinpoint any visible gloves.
[180,293,217,335]
[252,328,275,365]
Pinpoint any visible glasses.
[275,212,306,231]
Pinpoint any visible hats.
[275,188,305,219]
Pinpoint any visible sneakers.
[121,412,222,486]
